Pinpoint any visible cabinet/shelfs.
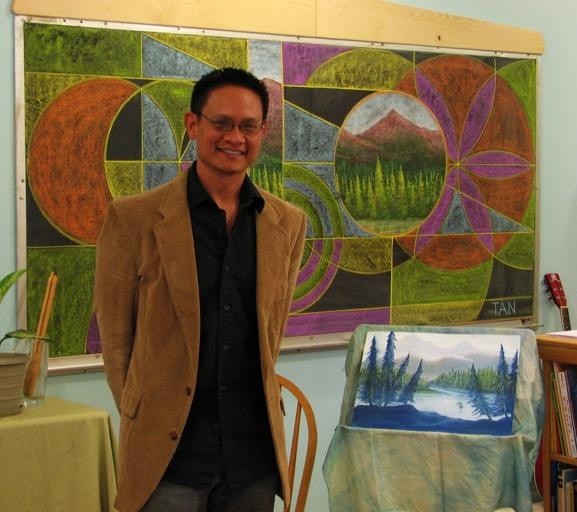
[534,332,577,512]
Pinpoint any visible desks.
[1,395,118,511]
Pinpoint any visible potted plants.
[0,262,54,418]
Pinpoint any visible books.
[550,360,577,512]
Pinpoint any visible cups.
[23,343,47,404]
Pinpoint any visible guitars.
[533,273,572,500]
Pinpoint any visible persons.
[93,67,307,512]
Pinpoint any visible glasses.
[200,114,266,136]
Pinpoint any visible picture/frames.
[9,13,542,379]
